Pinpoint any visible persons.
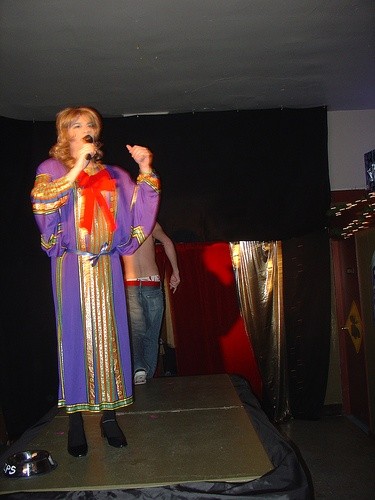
[32,105,160,457]
[121,221,181,383]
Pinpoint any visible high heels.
[67,412,89,457]
[99,410,128,448]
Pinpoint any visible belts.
[124,281,161,287]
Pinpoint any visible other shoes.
[134,369,147,385]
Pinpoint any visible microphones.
[83,135,93,161]
